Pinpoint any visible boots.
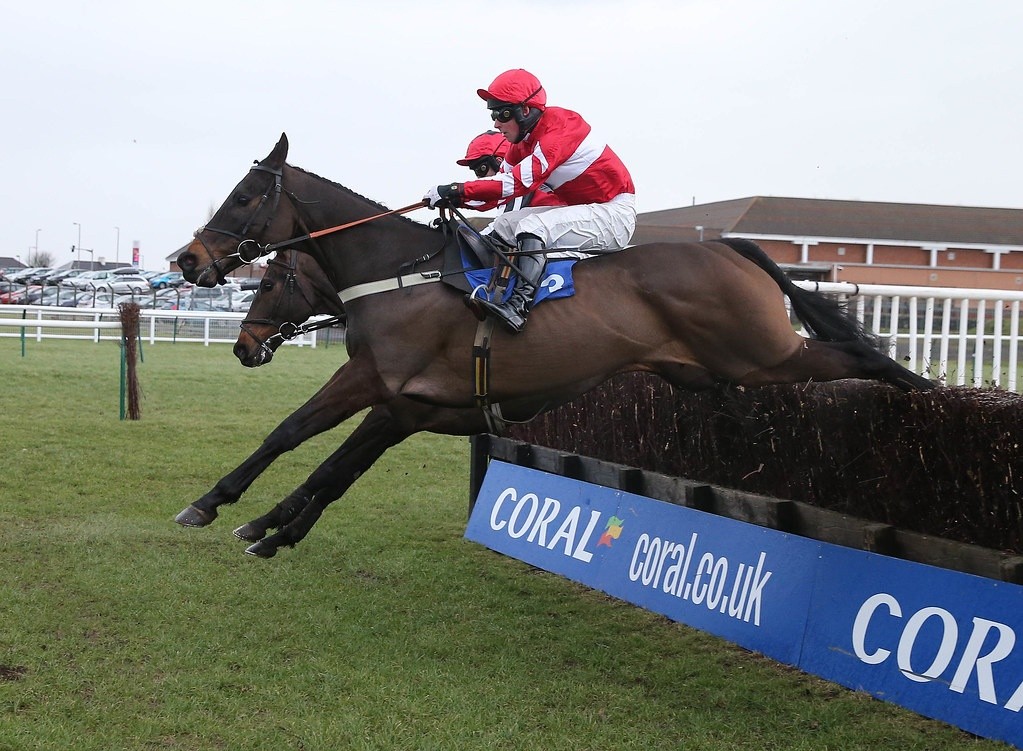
[475,238,547,335]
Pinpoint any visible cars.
[138,270,181,290]
[0,268,64,305]
[110,288,175,309]
[168,275,263,313]
[31,290,119,309]
[163,298,186,310]
[306,313,340,328]
[58,267,152,294]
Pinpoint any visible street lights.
[73,223,80,268]
[29,247,34,268]
[15,255,21,267]
[35,228,41,267]
[695,226,704,241]
[114,226,119,269]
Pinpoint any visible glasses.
[470,156,493,179]
[491,108,519,123]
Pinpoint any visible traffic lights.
[72,245,75,252]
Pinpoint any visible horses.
[172,131,941,561]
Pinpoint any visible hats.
[457,129,510,168]
[477,68,547,112]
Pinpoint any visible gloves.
[422,183,462,211]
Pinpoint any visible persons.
[422,69,638,335]
